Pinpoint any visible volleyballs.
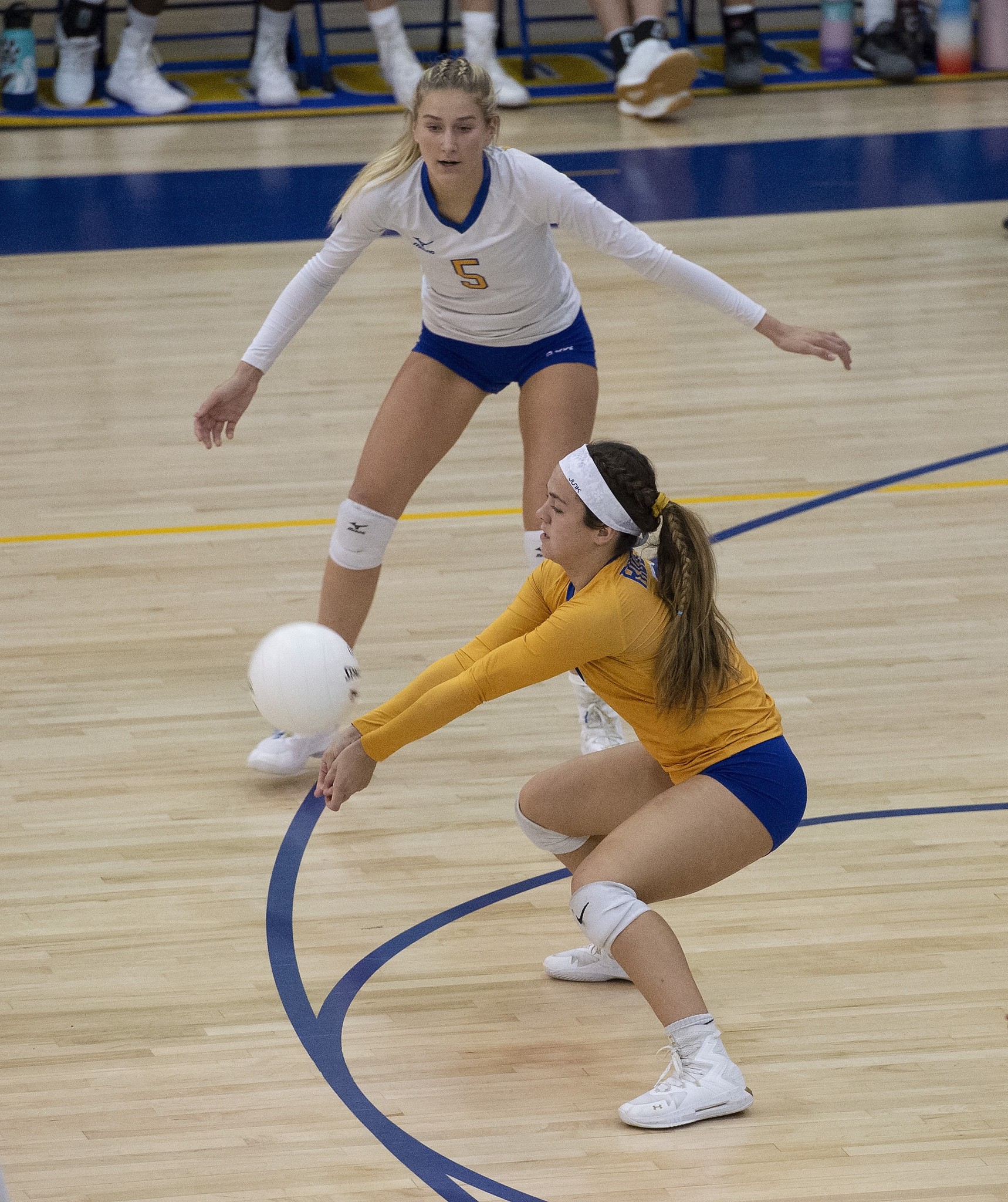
[245,620,361,738]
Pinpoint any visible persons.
[51,0,917,121]
[193,57,853,779]
[312,441,807,1130]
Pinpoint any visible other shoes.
[105,28,191,113]
[721,11,764,87]
[853,18,919,84]
[374,23,423,102]
[53,21,101,107]
[461,23,531,106]
[616,38,695,119]
[247,21,302,106]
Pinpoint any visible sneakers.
[578,698,623,757]
[617,1024,755,1128]
[542,941,632,983]
[249,721,346,771]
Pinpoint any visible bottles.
[820,0,853,68]
[0,2,38,110]
[935,1,978,75]
